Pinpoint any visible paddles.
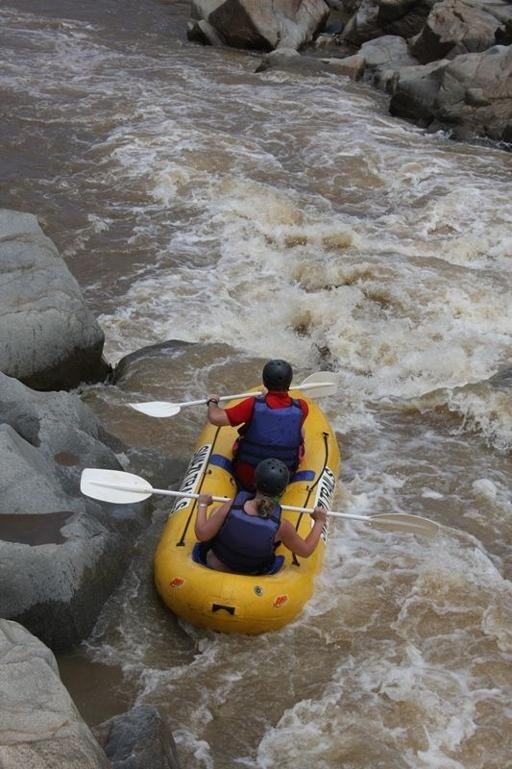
[79,467,439,537]
[125,371,340,418]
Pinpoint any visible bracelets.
[207,398,218,405]
[198,503,208,508]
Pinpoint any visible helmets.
[261,358,293,393]
[252,457,290,498]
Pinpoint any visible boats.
[152,384,345,634]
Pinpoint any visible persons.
[206,359,309,491]
[193,456,328,576]
